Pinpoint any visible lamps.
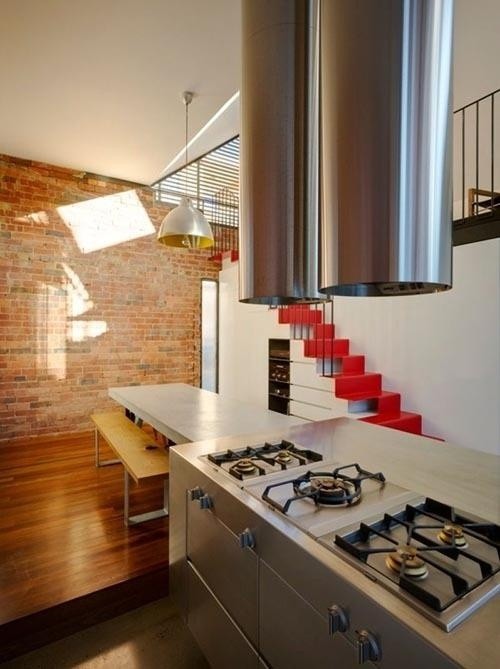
[154,90,214,251]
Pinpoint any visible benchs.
[89,410,173,528]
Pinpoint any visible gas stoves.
[196,437,500,633]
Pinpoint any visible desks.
[108,381,313,477]
